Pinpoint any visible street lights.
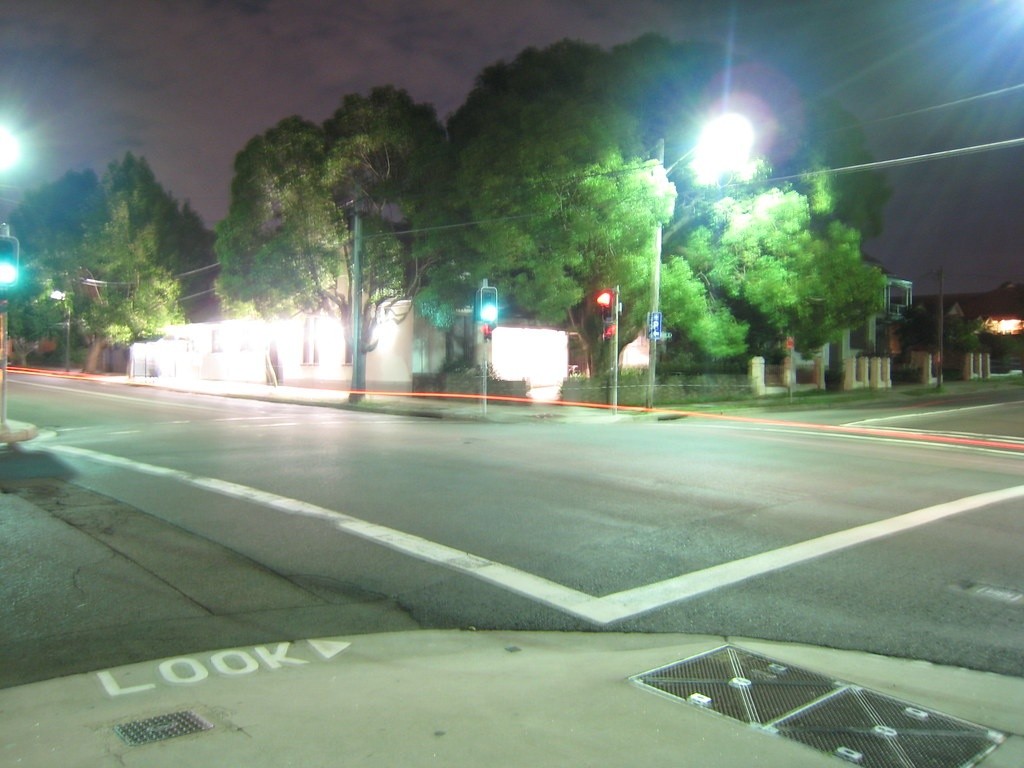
[646,113,752,407]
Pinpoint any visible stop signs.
[598,290,614,322]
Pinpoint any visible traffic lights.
[603,324,614,341]
[0,292,13,314]
[483,323,493,339]
[0,235,19,289]
[480,288,497,320]
